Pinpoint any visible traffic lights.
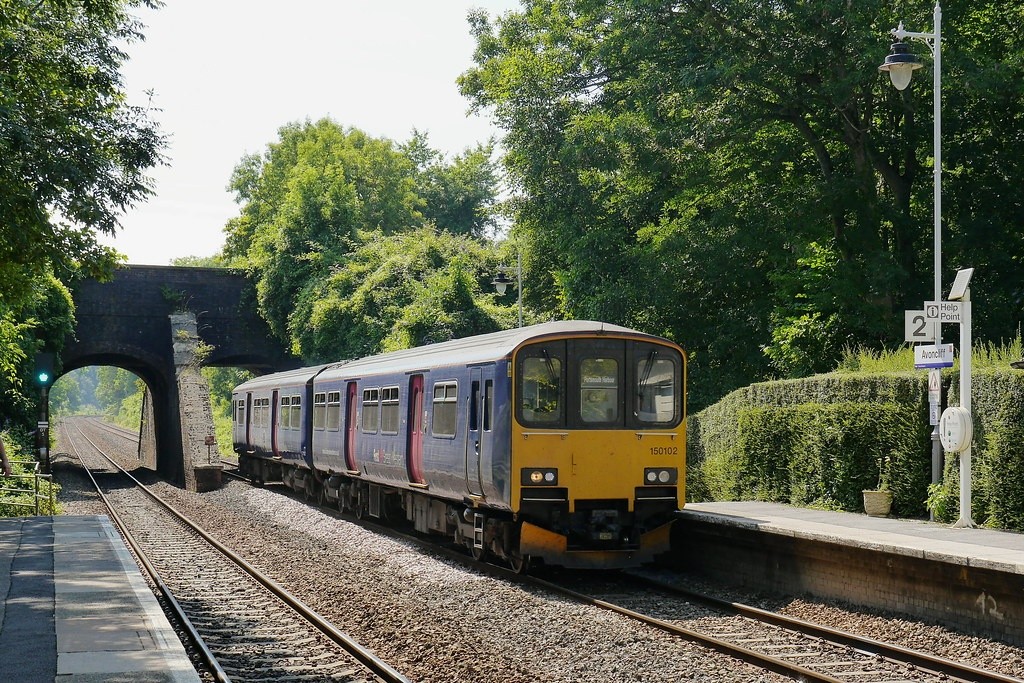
[36,353,53,387]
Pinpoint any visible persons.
[0,438,10,476]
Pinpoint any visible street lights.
[876,20,941,522]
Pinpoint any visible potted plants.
[862,473,892,518]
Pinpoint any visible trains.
[231,319,689,574]
